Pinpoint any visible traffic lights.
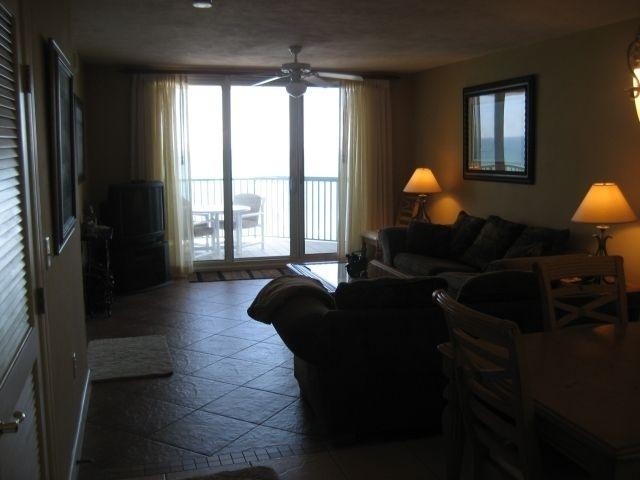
[434,209,574,274]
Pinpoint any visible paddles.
[174,465,281,479]
[190,264,296,285]
[87,330,175,384]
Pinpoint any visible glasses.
[401,166,443,224]
[622,23,640,127]
[561,179,639,280]
[284,78,309,99]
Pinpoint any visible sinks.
[258,208,577,447]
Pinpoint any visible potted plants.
[73,91,85,184]
[461,74,536,185]
[45,37,81,257]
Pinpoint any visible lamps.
[361,192,418,262]
[182,197,217,259]
[217,191,267,258]
[431,284,558,480]
[532,251,629,335]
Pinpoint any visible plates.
[80,220,118,321]
[109,239,176,298]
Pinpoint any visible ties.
[108,181,167,245]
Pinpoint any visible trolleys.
[253,42,365,87]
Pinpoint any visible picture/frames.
[192,202,252,260]
[435,318,640,479]
